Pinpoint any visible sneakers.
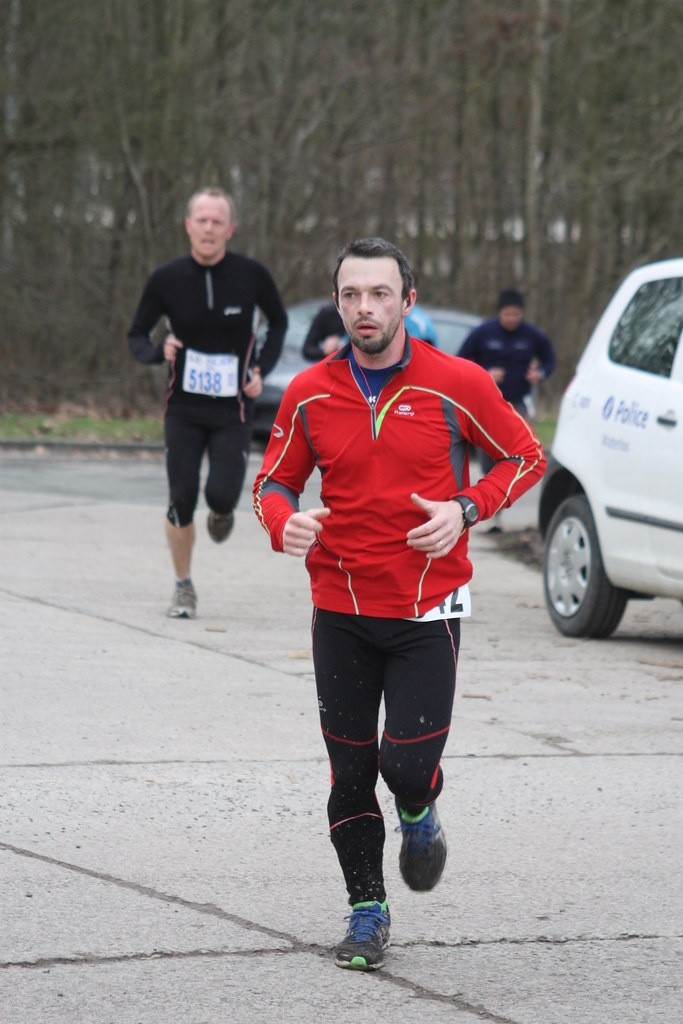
[395,801,447,892]
[167,584,198,619]
[206,511,235,543]
[332,897,391,971]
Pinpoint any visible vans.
[539,260,683,641]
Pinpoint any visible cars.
[245,295,489,450]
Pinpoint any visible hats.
[496,288,524,308]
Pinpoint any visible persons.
[300,270,438,361]
[127,188,289,619]
[455,289,558,533]
[251,237,548,970]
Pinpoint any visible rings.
[440,540,443,545]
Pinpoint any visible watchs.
[453,495,480,529]
